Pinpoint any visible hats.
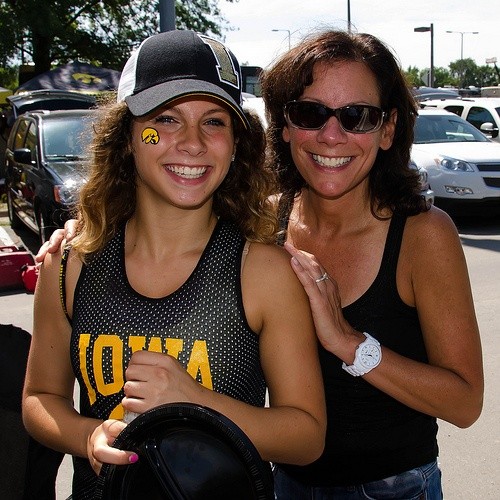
[116,30,253,135]
[94,401,274,500]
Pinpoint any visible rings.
[314,272,329,283]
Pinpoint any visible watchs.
[342,332,382,376]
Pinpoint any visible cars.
[4,88,99,247]
[409,108,500,207]
[410,86,500,141]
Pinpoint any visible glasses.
[284,100,388,134]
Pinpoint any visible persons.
[33,33,484,500]
[21,31,327,500]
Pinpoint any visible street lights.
[271,28,291,52]
[412,23,434,87]
[446,30,479,87]
